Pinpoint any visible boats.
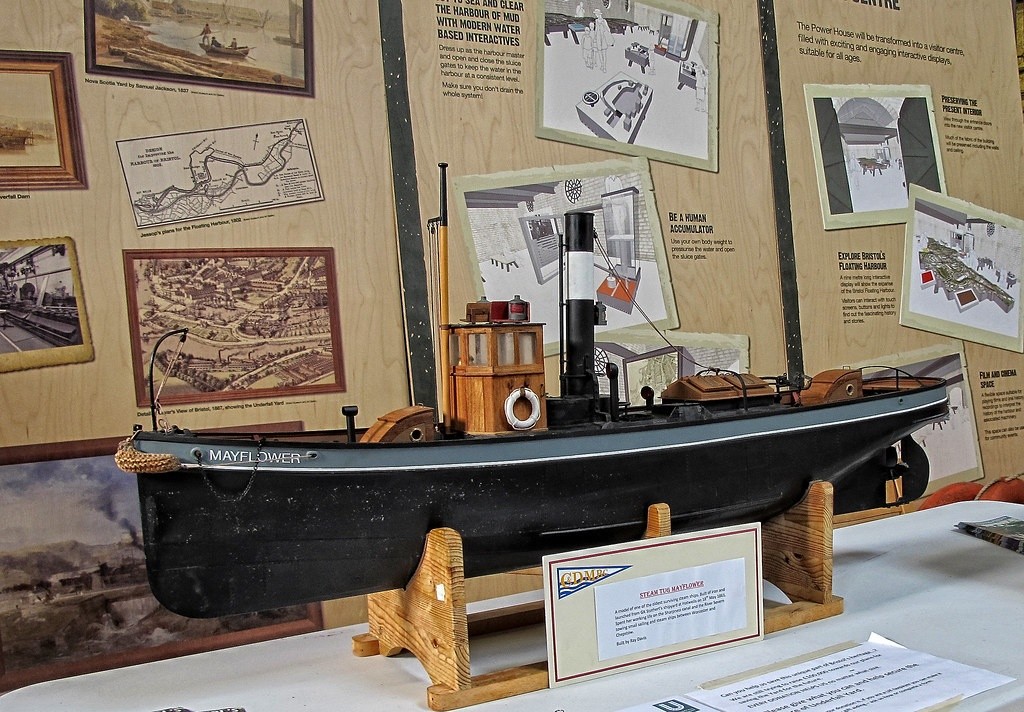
[0,124,34,148]
[116,163,951,619]
[197,42,250,58]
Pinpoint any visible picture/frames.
[121,246,346,409]
[83,0,314,98]
[0,48,88,192]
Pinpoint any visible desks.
[0,500,1024,712]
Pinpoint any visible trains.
[1,242,70,305]
